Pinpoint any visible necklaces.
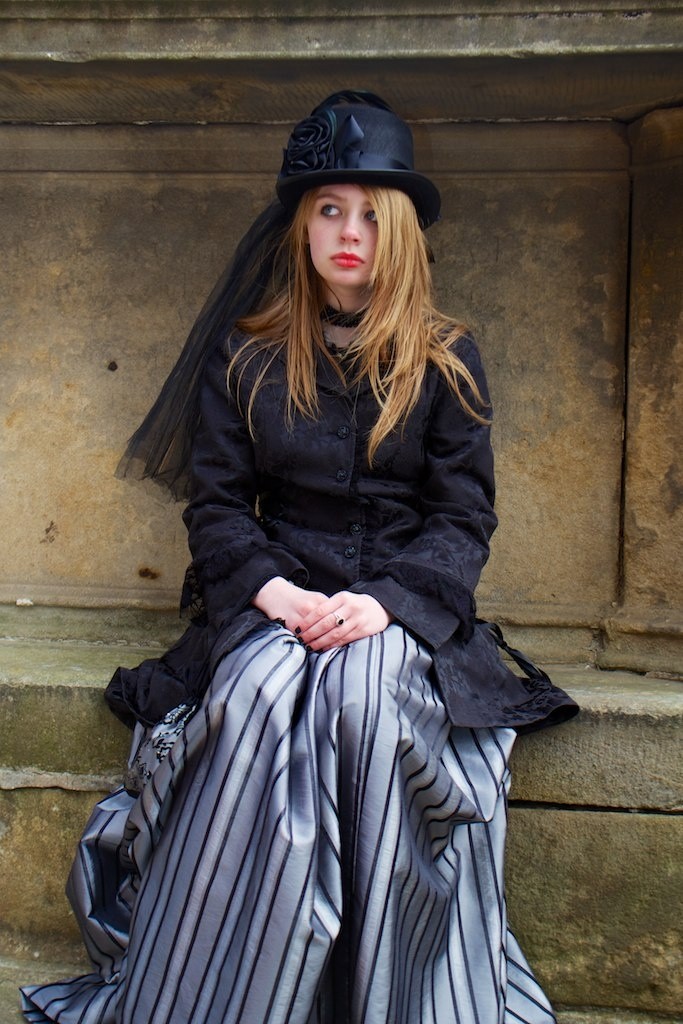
[319,305,365,329]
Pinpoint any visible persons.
[19,89,581,1024]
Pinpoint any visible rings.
[332,613,344,626]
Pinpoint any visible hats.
[275,89,440,233]
